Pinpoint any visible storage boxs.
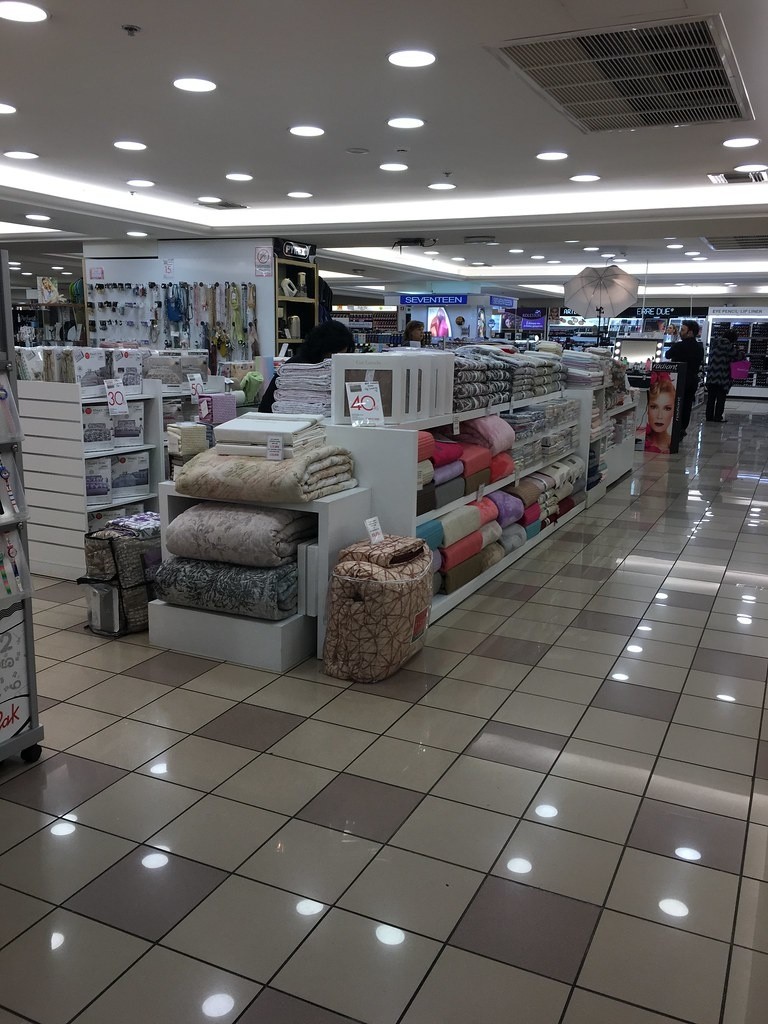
[429,355,435,417]
[13,347,44,379]
[330,354,403,424]
[88,507,125,532]
[124,504,144,516]
[108,452,150,498]
[401,352,416,421]
[112,402,145,446]
[83,406,114,451]
[298,539,317,617]
[86,459,112,505]
[434,356,444,415]
[415,354,429,419]
[106,350,143,394]
[40,349,63,382]
[61,349,108,398]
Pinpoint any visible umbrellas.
[563,265,638,347]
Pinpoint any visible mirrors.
[614,338,662,362]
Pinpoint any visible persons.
[551,307,559,319]
[665,320,704,437]
[258,320,356,413]
[645,379,676,455]
[477,308,486,337]
[668,324,677,335]
[504,315,514,328]
[705,329,746,423]
[41,277,59,303]
[403,320,426,348]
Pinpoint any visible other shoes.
[706,417,727,423]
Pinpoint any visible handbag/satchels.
[730,359,751,379]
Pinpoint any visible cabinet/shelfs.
[0,250,768,762]
